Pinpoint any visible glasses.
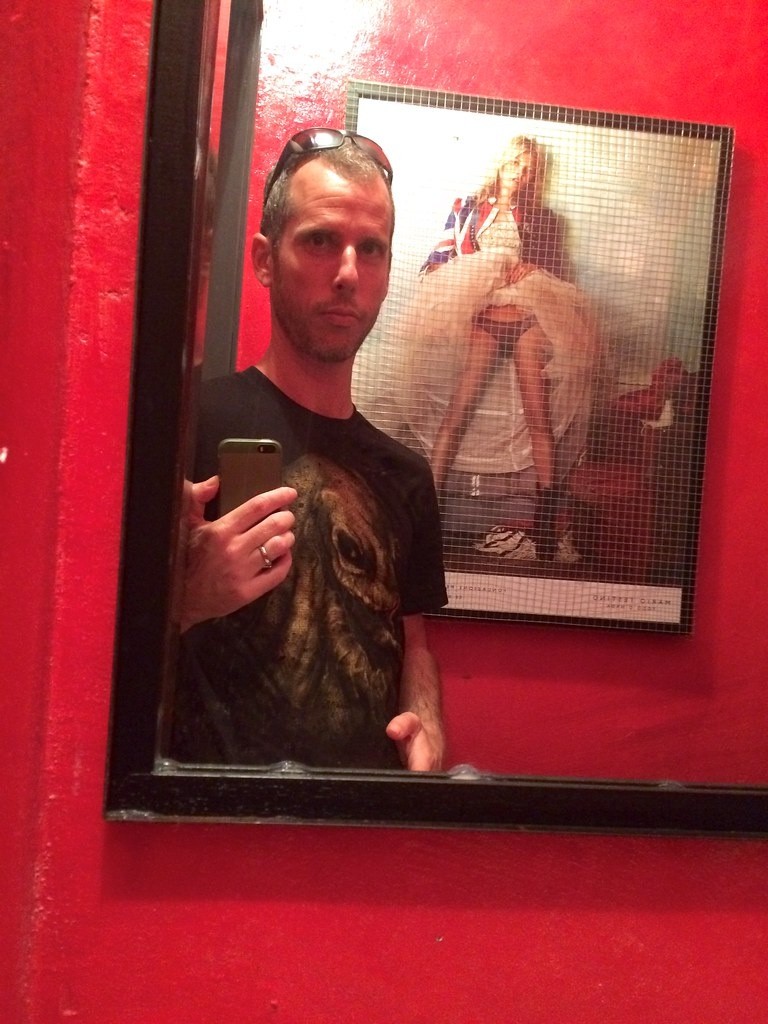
[265,128,393,205]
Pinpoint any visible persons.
[167,126,447,770]
[429,136,568,562]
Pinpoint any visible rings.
[257,545,272,569]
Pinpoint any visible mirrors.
[104,0,768,843]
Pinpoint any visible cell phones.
[218,437,283,519]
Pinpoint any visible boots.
[535,479,557,561]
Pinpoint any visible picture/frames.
[341,74,734,640]
